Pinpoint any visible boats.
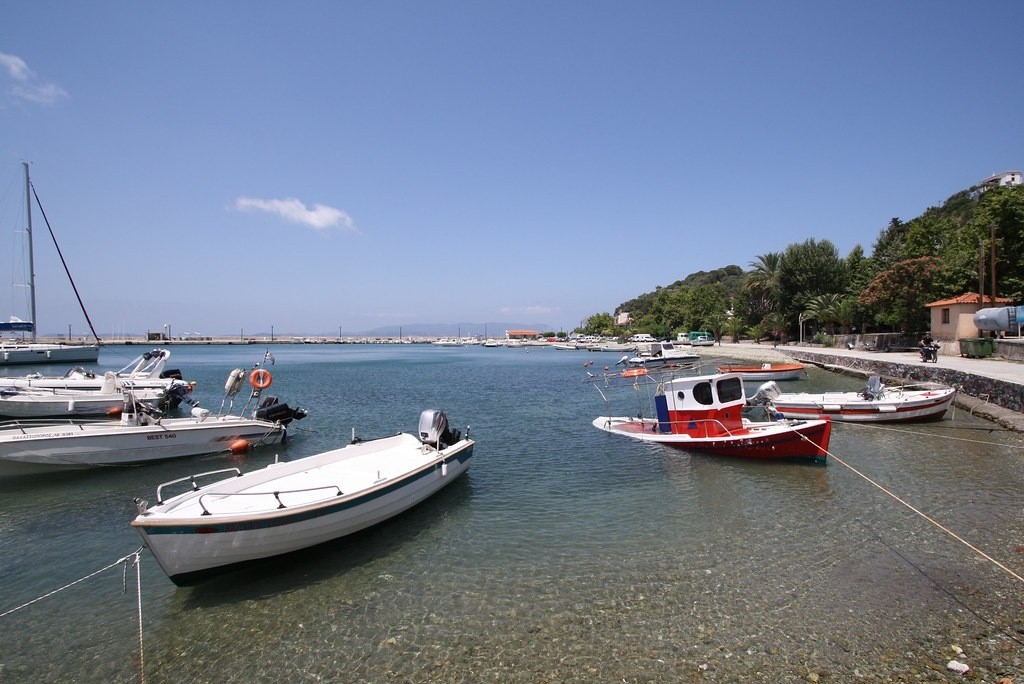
[0,347,308,476]
[592,340,956,464]
[131,409,475,588]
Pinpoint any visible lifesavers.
[225,368,245,396]
[250,368,272,389]
[621,369,647,377]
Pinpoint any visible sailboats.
[0,158,102,365]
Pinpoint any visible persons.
[772,329,781,348]
[919,331,933,358]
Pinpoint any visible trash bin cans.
[958,337,996,359]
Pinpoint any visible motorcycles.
[920,340,940,363]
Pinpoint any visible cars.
[539,332,715,347]
